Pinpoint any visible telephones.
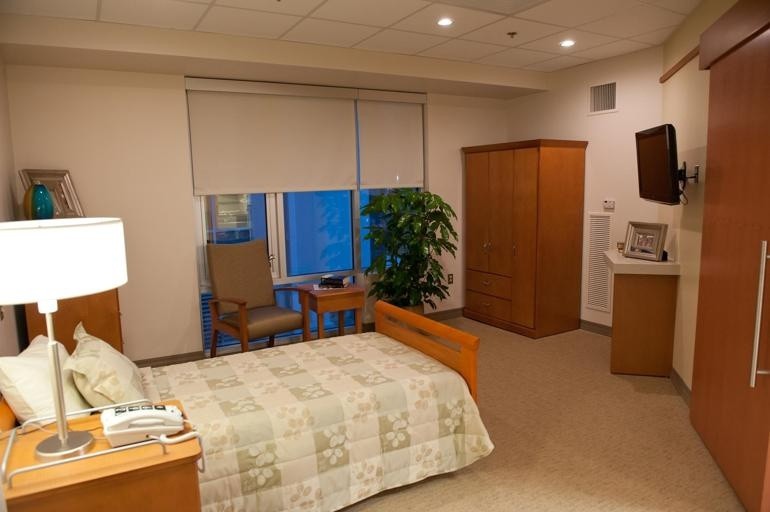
[101,404,184,447]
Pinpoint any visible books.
[318,272,350,288]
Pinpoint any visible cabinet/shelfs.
[23,287,124,356]
[464,267,514,332]
[602,249,681,378]
[459,143,513,277]
[514,138,590,342]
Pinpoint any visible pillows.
[1,334,93,429]
[61,319,148,414]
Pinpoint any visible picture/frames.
[18,168,86,219]
[621,221,669,264]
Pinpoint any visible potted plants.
[358,186,461,316]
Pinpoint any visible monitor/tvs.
[635,123,686,206]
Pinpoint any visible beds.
[1,299,496,510]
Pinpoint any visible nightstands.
[1,397,207,511]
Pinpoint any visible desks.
[298,283,369,343]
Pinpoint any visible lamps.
[2,216,130,468]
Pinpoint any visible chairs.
[206,238,312,358]
